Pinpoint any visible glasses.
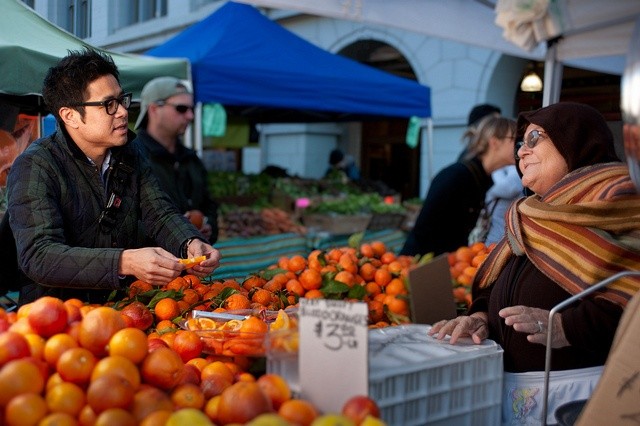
[514,129,548,160]
[164,101,194,114]
[69,92,134,115]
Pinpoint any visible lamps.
[520,61,543,92]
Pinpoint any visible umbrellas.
[140,0,436,199]
[0,0,196,185]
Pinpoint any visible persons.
[465,104,529,250]
[4,44,220,310]
[129,74,219,243]
[429,103,640,425]
[400,116,518,254]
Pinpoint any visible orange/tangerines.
[268,240,499,354]
[0,255,268,426]
[123,256,284,314]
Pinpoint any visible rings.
[536,320,544,331]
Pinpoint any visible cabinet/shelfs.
[198,171,422,282]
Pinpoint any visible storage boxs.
[275,323,503,426]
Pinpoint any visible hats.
[133,75,194,132]
